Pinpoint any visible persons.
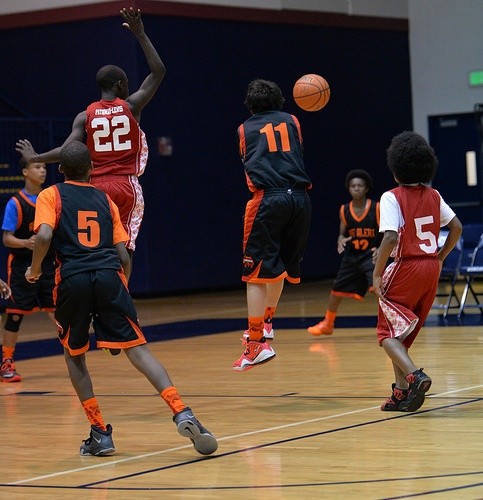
[306,170,384,336]
[0,279,12,299]
[371,132,463,412]
[0,161,60,383]
[25,140,218,455]
[233,79,313,373]
[17,7,167,287]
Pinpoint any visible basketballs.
[293,74,330,111]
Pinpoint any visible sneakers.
[405,367,432,411]
[307,320,334,336]
[173,406,218,455]
[380,382,408,411]
[242,315,275,339]
[0,358,22,382]
[80,424,116,456]
[231,336,277,371]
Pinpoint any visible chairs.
[430,234,483,318]
